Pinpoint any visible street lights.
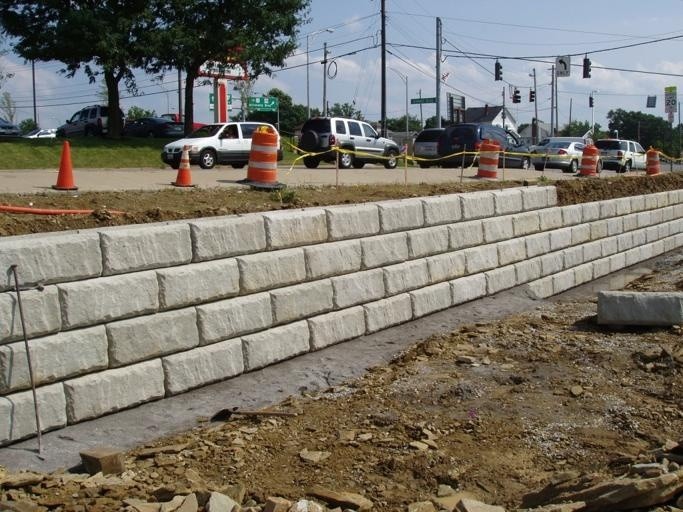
[495,63,502,80]
[583,59,591,78]
[530,91,535,102]
[592,89,600,134]
[307,27,334,118]
[590,97,593,107]
[513,91,520,103]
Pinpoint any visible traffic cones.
[52,141,78,190]
[171,144,195,186]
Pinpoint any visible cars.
[0,105,208,139]
[298,117,399,168]
[161,122,282,168]
[414,123,647,173]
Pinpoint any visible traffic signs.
[665,87,677,112]
